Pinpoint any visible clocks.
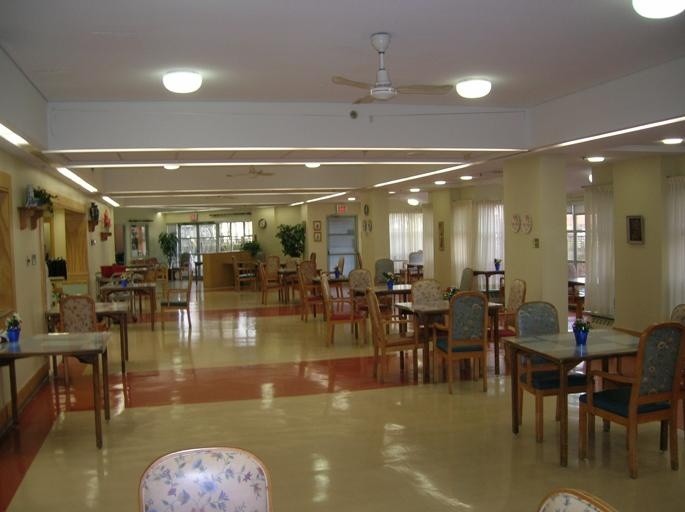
[258,218,267,229]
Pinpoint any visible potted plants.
[157,232,178,281]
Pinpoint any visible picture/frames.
[313,220,321,231]
[314,232,323,242]
[625,215,646,246]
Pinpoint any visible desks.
[501,326,649,468]
[392,298,504,386]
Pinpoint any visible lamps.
[161,72,204,95]
[456,79,492,100]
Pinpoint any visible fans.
[331,32,454,106]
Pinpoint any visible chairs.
[0,253,196,452]
[576,319,683,479]
[231,256,504,348]
[362,286,421,386]
[431,288,490,395]
[657,302,685,455]
[138,445,273,512]
[513,299,599,442]
[534,487,616,512]
